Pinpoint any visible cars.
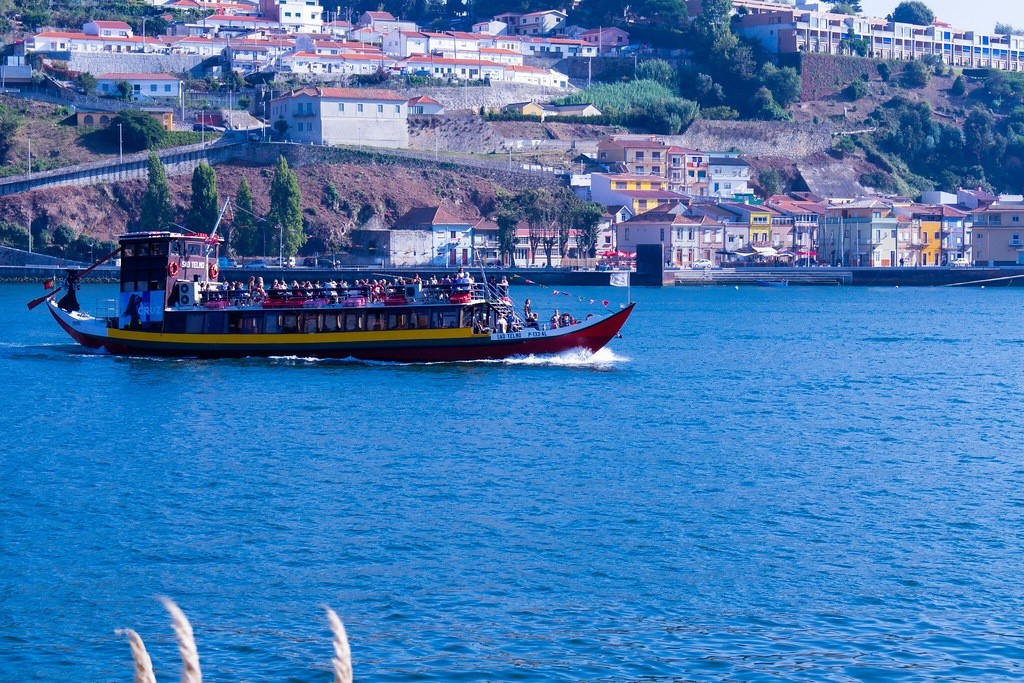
[693,259,713,268]
[948,258,970,268]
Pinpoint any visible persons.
[899,257,904,267]
[720,259,842,267]
[595,259,620,271]
[200,268,576,335]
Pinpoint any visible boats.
[26,198,639,362]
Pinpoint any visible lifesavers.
[559,313,575,328]
[168,260,179,277]
[209,264,221,279]
